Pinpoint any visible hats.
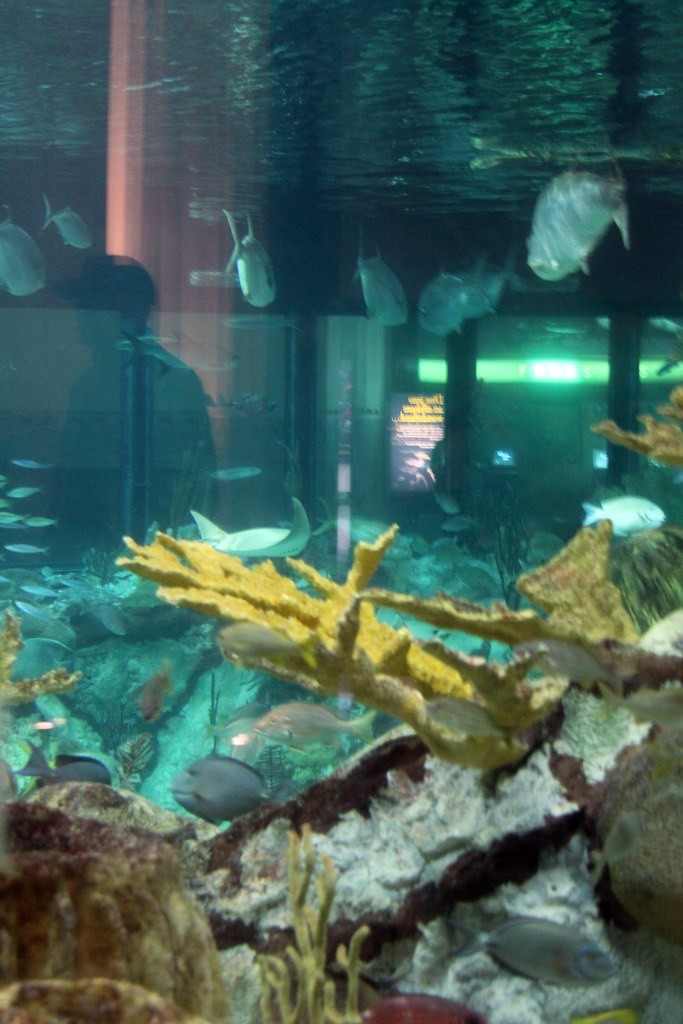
[52,255,160,302]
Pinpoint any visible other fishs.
[542,313,681,378]
[190,466,312,560]
[40,191,94,250]
[580,493,665,540]
[413,485,574,600]
[110,328,240,381]
[0,204,48,297]
[330,913,641,1024]
[224,392,279,418]
[1,734,112,802]
[353,239,410,327]
[226,313,304,334]
[1,455,128,640]
[415,247,528,336]
[522,148,631,281]
[221,206,284,307]
[313,495,413,563]
[114,620,683,828]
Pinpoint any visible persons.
[54,253,216,574]
